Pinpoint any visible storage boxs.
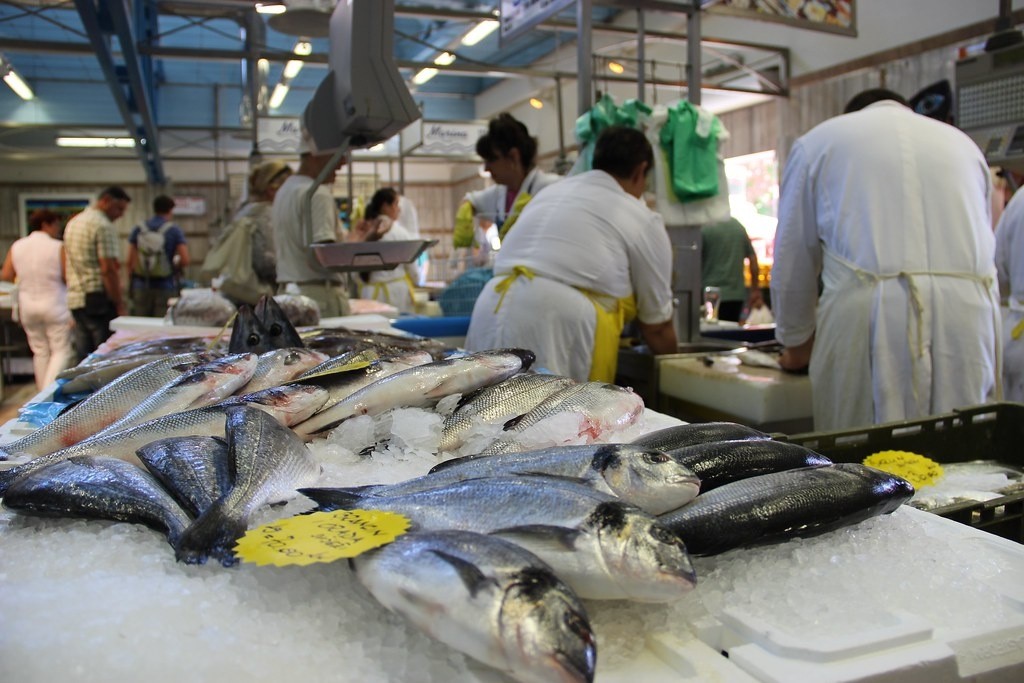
[765,401,1024,544]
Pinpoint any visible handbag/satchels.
[11,285,21,323]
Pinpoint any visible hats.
[249,160,287,197]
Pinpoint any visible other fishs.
[736,339,783,369]
[298,295,319,325]
[272,294,300,324]
[309,349,433,414]
[56,336,206,394]
[292,422,914,683]
[0,383,331,496]
[357,373,644,456]
[262,298,301,347]
[134,435,231,518]
[0,352,212,457]
[73,352,258,445]
[291,348,535,439]
[1,455,240,569]
[229,302,271,355]
[172,295,237,327]
[295,327,452,379]
[907,483,1024,523]
[172,405,323,565]
[232,347,330,397]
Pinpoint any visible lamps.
[1,59,34,102]
[408,6,499,86]
[267,35,312,109]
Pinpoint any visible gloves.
[498,192,534,245]
[452,202,480,250]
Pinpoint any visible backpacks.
[131,219,174,280]
[197,199,273,306]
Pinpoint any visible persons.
[453,113,569,248]
[768,89,1002,432]
[362,187,422,308]
[702,216,759,323]
[129,194,190,317]
[230,153,350,318]
[989,164,1024,405]
[0,183,132,391]
[463,125,678,383]
